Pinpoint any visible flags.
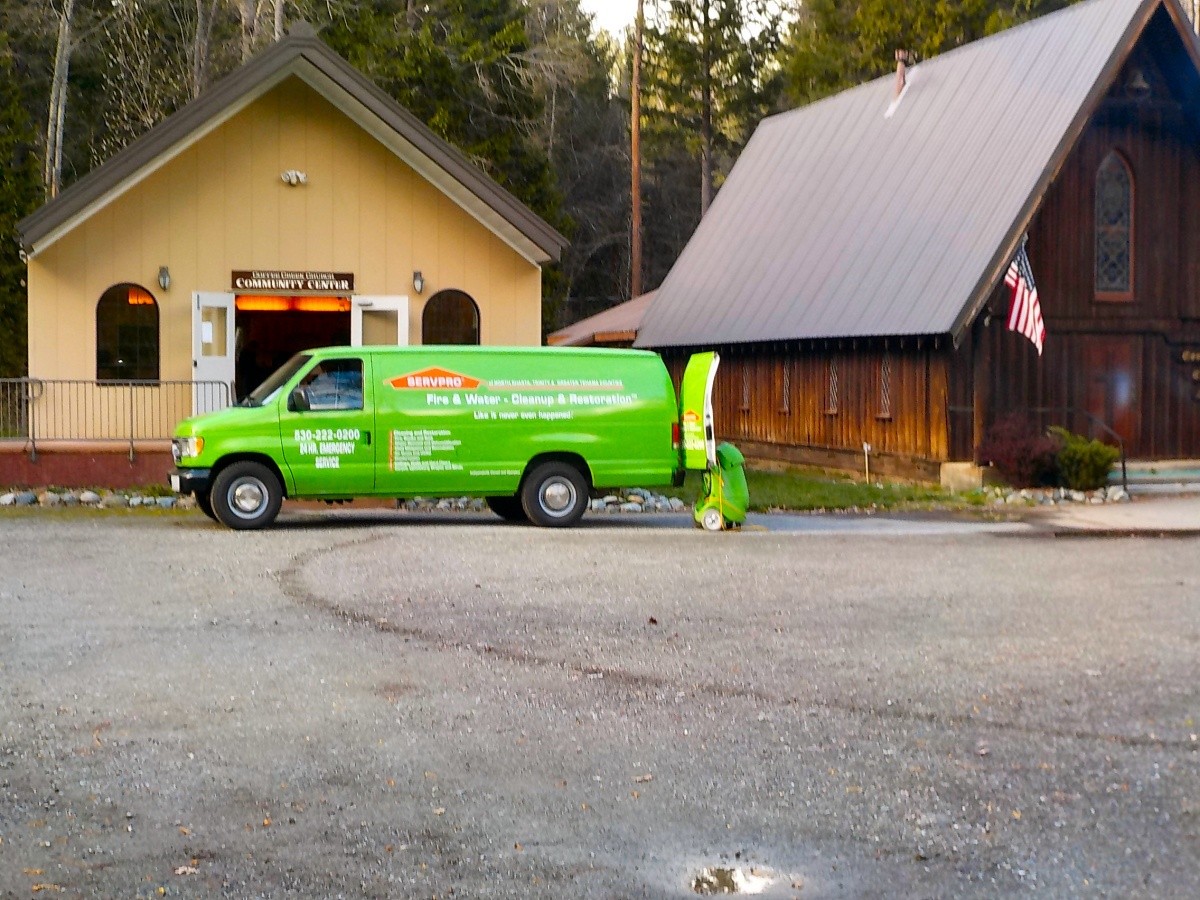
[1003,233,1046,358]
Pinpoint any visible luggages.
[693,442,749,532]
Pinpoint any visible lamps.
[1125,69,1152,99]
[158,265,169,290]
[412,271,425,294]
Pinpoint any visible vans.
[171,345,720,531]
